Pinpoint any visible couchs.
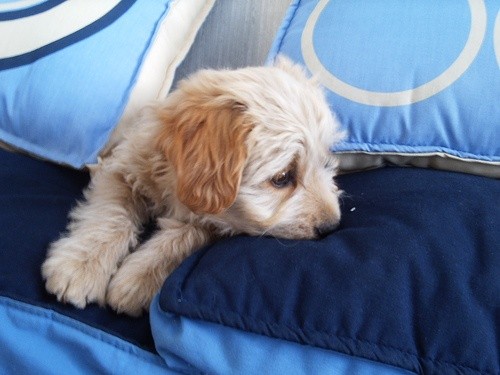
[0,1,499,375]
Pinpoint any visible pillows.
[0,1,216,180]
[266,1,500,180]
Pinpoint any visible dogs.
[41,53,350,318]
